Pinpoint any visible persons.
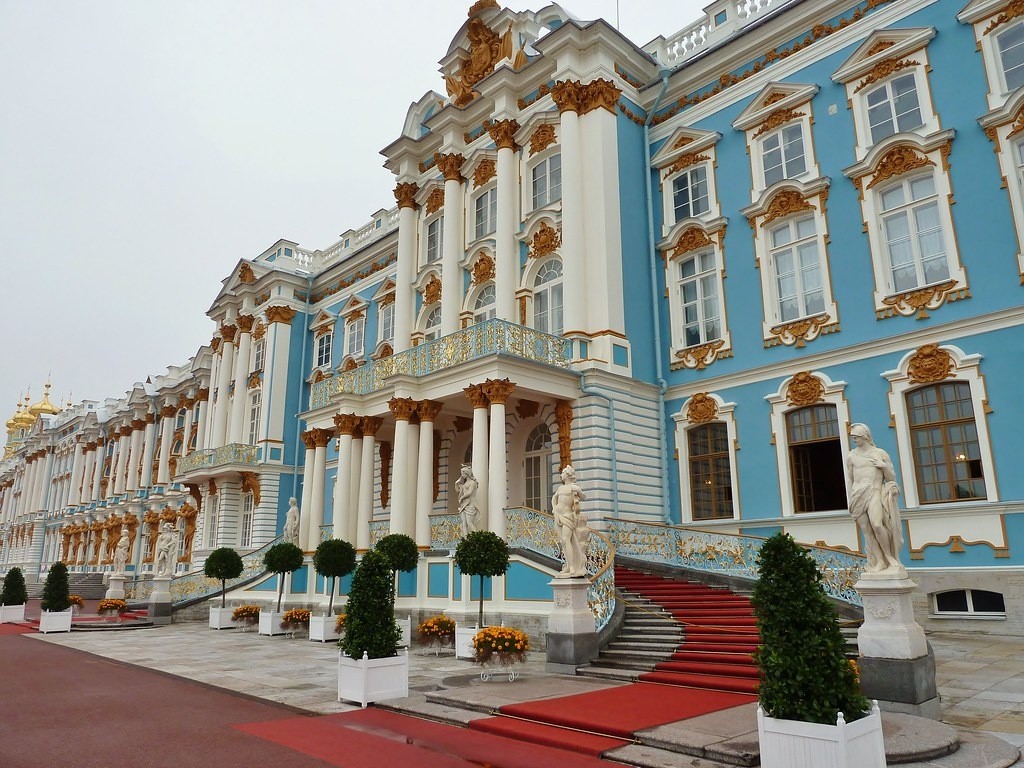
[454,465,480,537]
[62,501,195,550]
[154,523,177,577]
[552,465,586,578]
[111,529,129,577]
[284,497,300,546]
[845,422,908,573]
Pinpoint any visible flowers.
[279,607,310,631]
[417,613,456,648]
[231,605,260,626]
[849,660,860,684]
[334,614,347,634]
[69,596,85,606]
[472,625,530,667]
[97,599,128,617]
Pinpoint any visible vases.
[106,610,119,617]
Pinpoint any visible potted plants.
[454,530,511,659]
[309,538,358,642]
[336,547,408,708]
[749,531,886,768]
[205,547,244,630]
[376,533,420,647]
[39,561,72,633]
[258,542,304,636]
[0,566,28,624]
